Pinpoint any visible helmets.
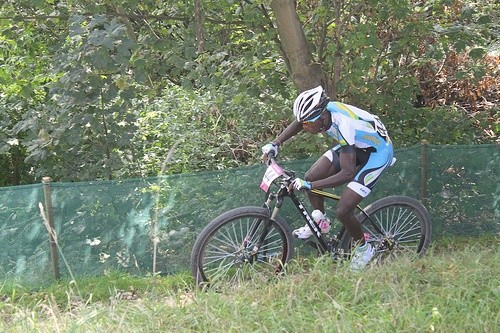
[292,85,330,123]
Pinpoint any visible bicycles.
[189,148,433,290]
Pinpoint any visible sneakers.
[349,244,375,272]
[291,210,331,239]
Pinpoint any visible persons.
[261,85,394,270]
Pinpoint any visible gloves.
[261,142,278,157]
[291,177,313,191]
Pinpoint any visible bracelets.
[276,137,282,145]
